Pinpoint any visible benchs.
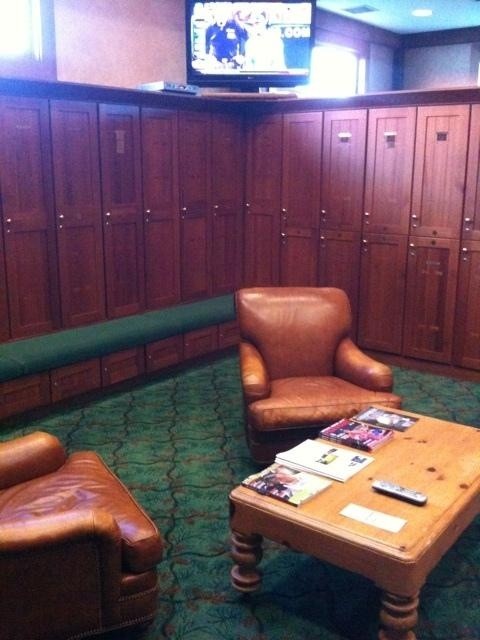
[0,293,240,433]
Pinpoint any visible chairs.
[233,286,402,467]
[1,430,162,639]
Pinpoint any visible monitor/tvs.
[184,0,316,93]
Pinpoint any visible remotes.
[371,479,428,506]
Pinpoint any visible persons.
[199,7,289,72]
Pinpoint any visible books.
[237,406,423,507]
